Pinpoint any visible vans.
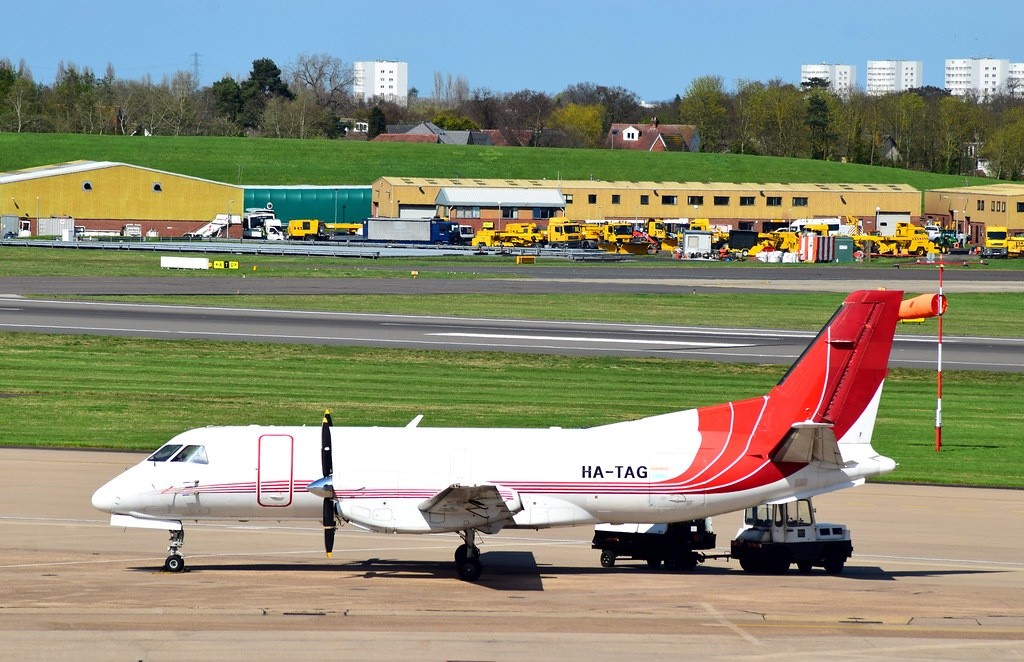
[787,218,841,238]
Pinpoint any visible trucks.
[731,497,853,575]
[243,208,324,240]
[452,216,729,254]
[724,216,1024,259]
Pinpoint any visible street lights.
[963,210,966,249]
[876,207,880,229]
[953,210,959,235]
[498,201,501,229]
[226,200,235,238]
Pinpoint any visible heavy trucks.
[367,218,454,250]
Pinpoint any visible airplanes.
[92,288,903,581]
